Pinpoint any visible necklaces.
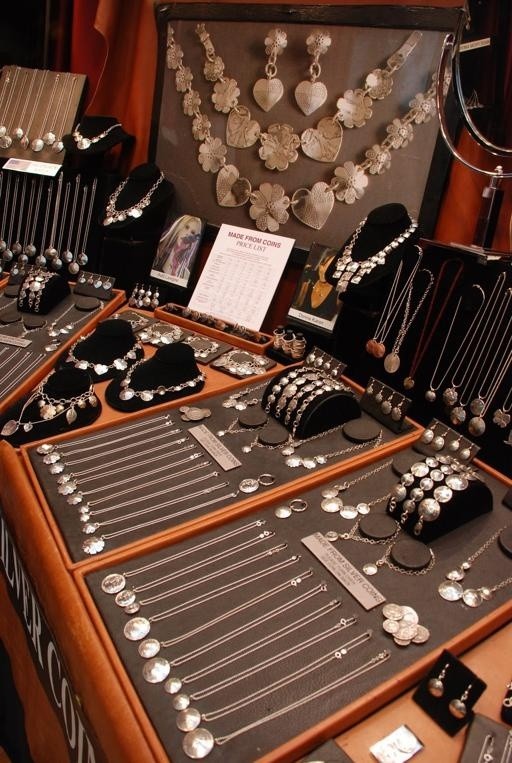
[1,17,512,762]
